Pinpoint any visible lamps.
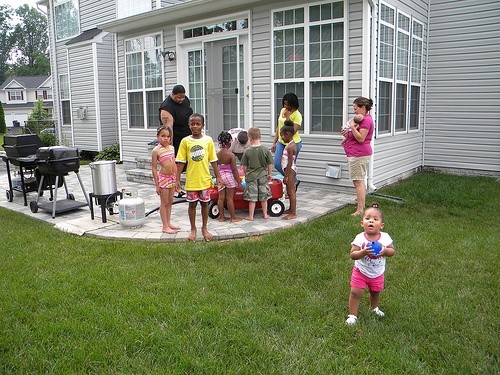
[161,50,177,61]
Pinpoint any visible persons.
[227,128,247,161]
[345,202,394,324]
[241,128,274,221]
[215,126,242,222]
[279,120,298,221]
[158,84,194,198]
[341,97,375,216]
[176,113,220,242]
[152,126,181,234]
[272,92,303,200]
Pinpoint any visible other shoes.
[345,314,357,325]
[369,307,385,317]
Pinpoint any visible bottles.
[367,240,381,255]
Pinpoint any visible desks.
[89,190,122,223]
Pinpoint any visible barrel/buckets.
[89,160,117,195]
